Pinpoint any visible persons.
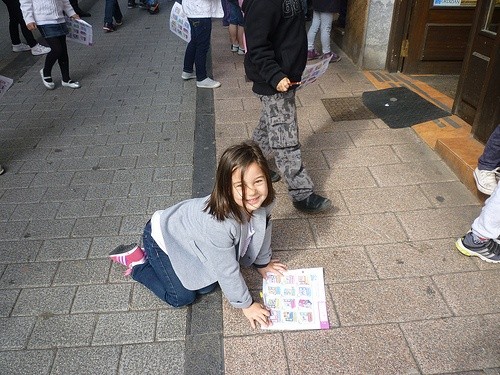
[105,142,290,331]
[454,180,500,265]
[180,0,246,90]
[244,0,334,214]
[0,163,5,175]
[2,0,51,56]
[299,0,311,22]
[127,0,148,9]
[472,123,500,196]
[63,0,91,17]
[102,0,123,31]
[19,0,81,89]
[306,0,343,62]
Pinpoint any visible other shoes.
[78,10,91,17]
[103,22,115,32]
[40,67,55,89]
[61,79,80,88]
[149,2,160,14]
[115,19,122,25]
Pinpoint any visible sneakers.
[473,167,500,195]
[307,49,322,59]
[238,47,245,54]
[12,41,31,52]
[196,77,221,88]
[108,243,146,275]
[139,3,147,9]
[269,169,280,182]
[31,43,52,55]
[455,227,500,263]
[141,248,150,259]
[231,44,239,51]
[321,51,342,62]
[292,192,332,214]
[128,3,135,8]
[182,71,196,79]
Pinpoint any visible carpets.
[362,87,451,128]
